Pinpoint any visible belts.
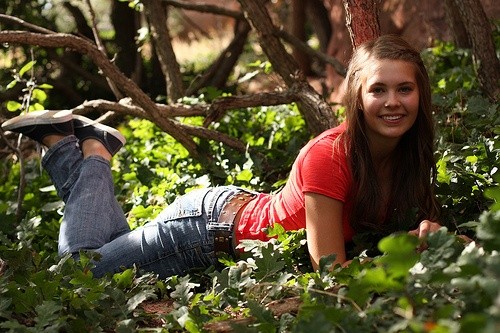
[214,190,257,274]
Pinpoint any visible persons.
[1,34,478,292]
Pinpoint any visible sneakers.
[71,114,127,157]
[1,109,74,145]
